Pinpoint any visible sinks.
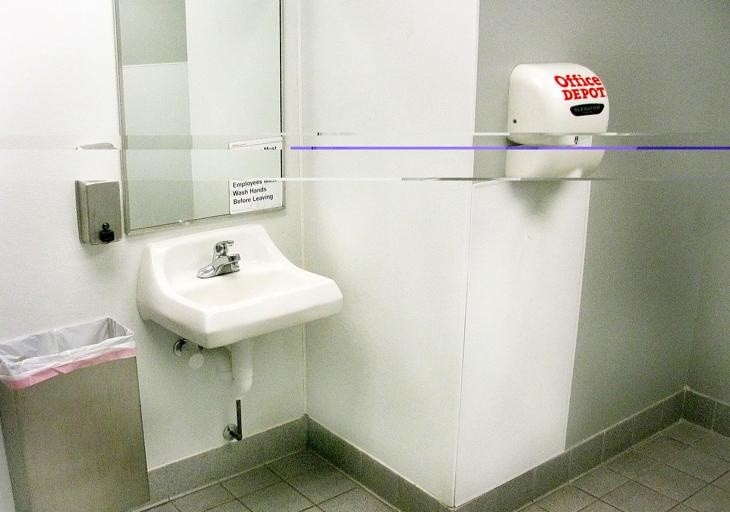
[136,223,344,350]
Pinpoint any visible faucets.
[196,241,241,279]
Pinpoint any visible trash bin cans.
[1,319,151,512]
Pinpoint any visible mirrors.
[112,1,287,235]
